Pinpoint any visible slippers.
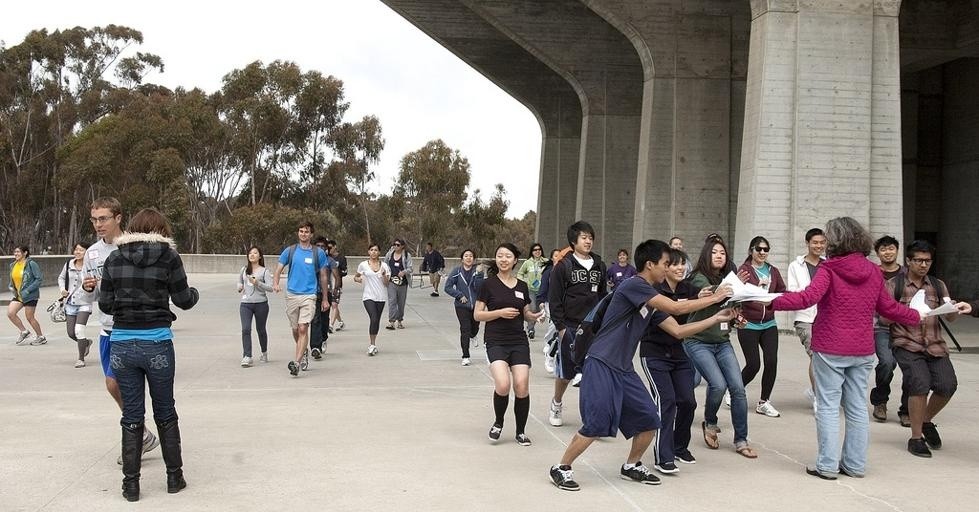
[736,445,758,458]
[702,421,721,450]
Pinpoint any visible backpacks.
[568,276,641,368]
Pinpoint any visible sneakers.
[620,463,660,487]
[653,456,678,475]
[725,389,731,408]
[116,430,161,466]
[755,401,781,418]
[461,329,582,387]
[547,462,580,491]
[804,387,943,482]
[430,291,439,297]
[75,340,91,367]
[30,336,48,345]
[678,449,699,467]
[487,422,503,441]
[240,315,407,376]
[514,434,532,447]
[549,397,563,427]
[15,328,33,345]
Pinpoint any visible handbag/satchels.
[46,291,69,322]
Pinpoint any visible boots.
[157,418,187,493]
[120,419,144,502]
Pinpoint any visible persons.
[4,243,47,347]
[78,194,161,466]
[98,209,200,504]
[56,241,97,370]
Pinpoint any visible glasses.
[913,258,933,266]
[752,246,770,252]
[89,215,115,222]
